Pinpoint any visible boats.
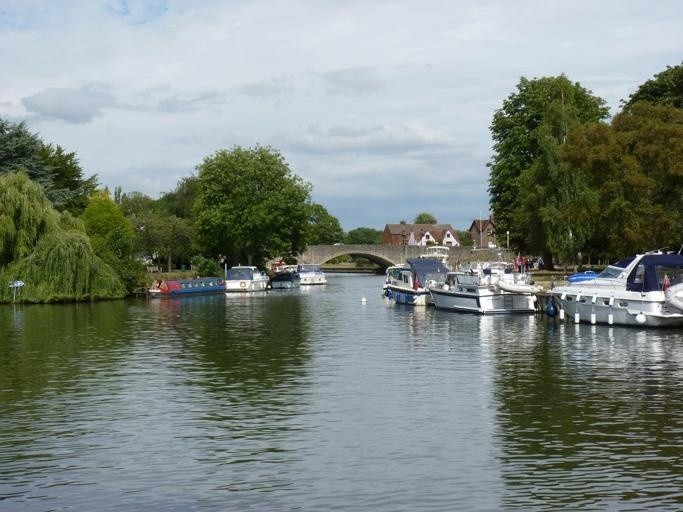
[149,258,329,296]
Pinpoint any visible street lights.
[507,230,510,249]
